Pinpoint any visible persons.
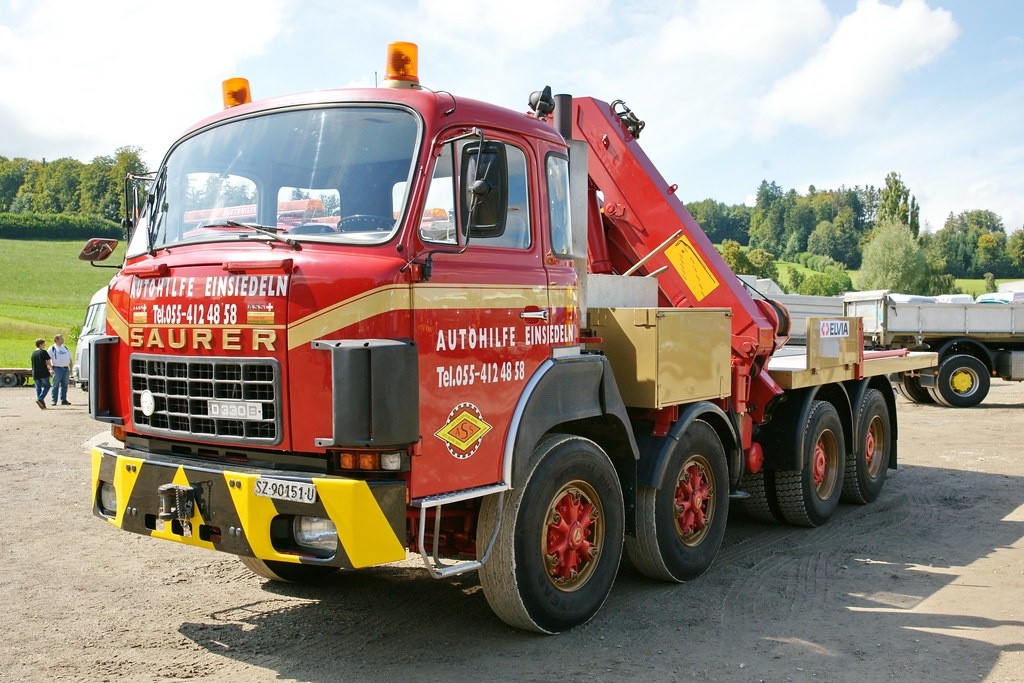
[31,338,53,409]
[47,334,73,406]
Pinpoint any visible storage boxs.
[586,307,733,410]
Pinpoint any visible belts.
[53,366,68,368]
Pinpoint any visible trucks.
[87,42,939,632]
[0,369,33,388]
[843,291,1022,409]
[738,275,843,343]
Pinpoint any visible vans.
[70,286,109,391]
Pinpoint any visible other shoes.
[36,399,46,409]
[51,401,56,406]
[61,400,71,405]
